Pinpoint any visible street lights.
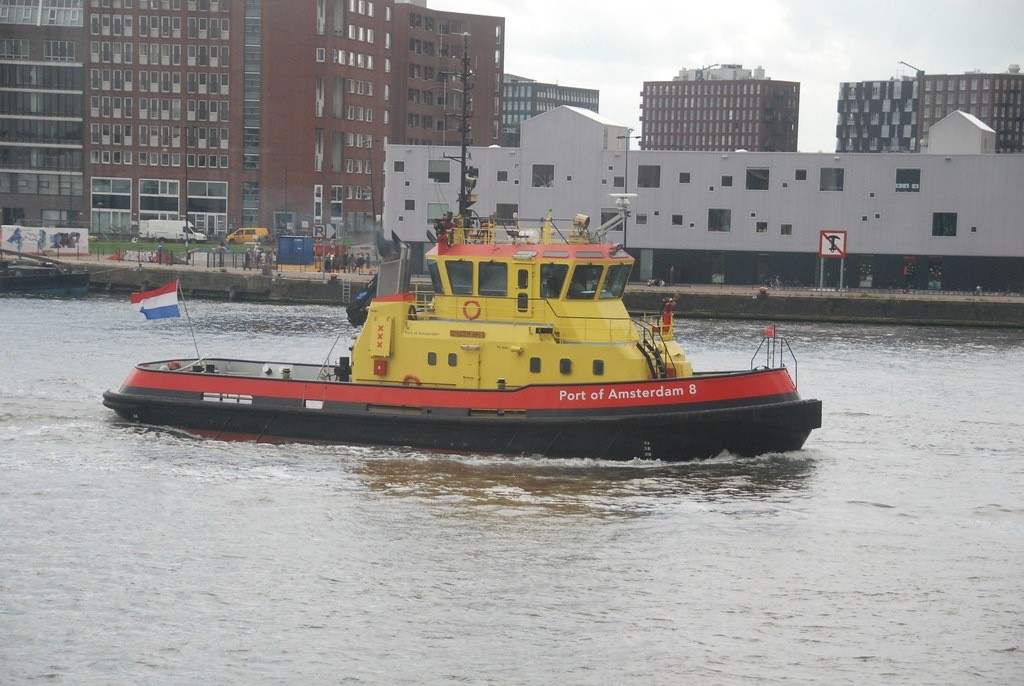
[615,134,643,250]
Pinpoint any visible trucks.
[147,219,208,245]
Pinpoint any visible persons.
[216,243,227,268]
[315,250,371,274]
[564,271,586,299]
[242,247,279,272]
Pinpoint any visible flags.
[130,279,181,320]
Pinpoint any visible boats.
[1,258,91,298]
[101,208,822,460]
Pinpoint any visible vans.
[225,227,269,244]
